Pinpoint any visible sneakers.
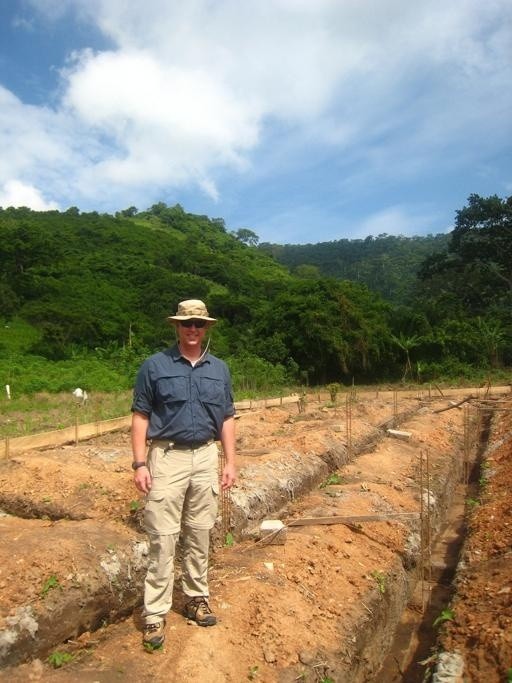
[183,596,216,626]
[144,619,166,649]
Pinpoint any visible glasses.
[181,320,205,328]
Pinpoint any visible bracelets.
[131,460,147,469]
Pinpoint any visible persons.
[128,298,241,653]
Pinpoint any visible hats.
[166,300,217,327]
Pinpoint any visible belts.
[176,440,206,448]
